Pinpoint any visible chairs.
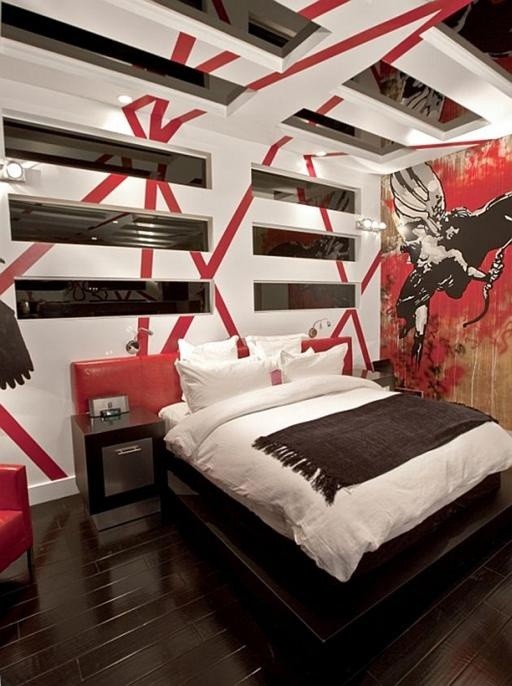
[0,461,35,581]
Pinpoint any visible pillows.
[177,334,240,403]
[172,348,274,413]
[278,341,348,384]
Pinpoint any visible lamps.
[354,215,388,234]
[1,157,27,185]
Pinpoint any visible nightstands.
[69,406,171,533]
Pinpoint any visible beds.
[70,337,512,653]
